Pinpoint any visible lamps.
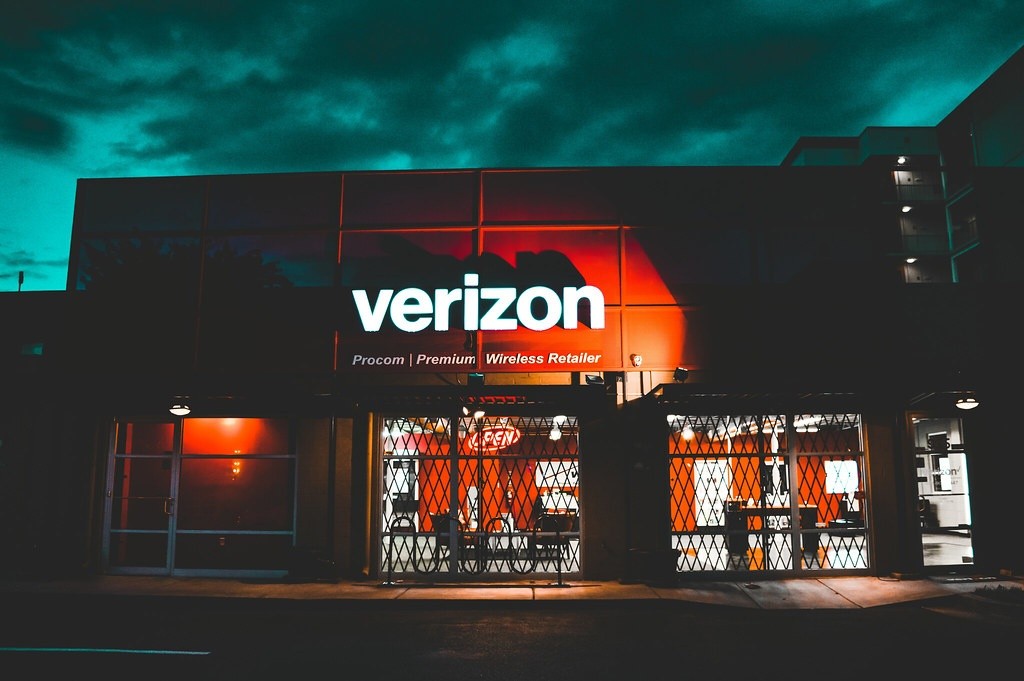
[467,372,485,389]
[672,367,688,384]
[955,393,979,409]
[168,397,191,415]
[549,423,562,440]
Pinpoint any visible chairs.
[429,512,479,562]
[525,511,577,557]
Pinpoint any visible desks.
[723,505,819,555]
